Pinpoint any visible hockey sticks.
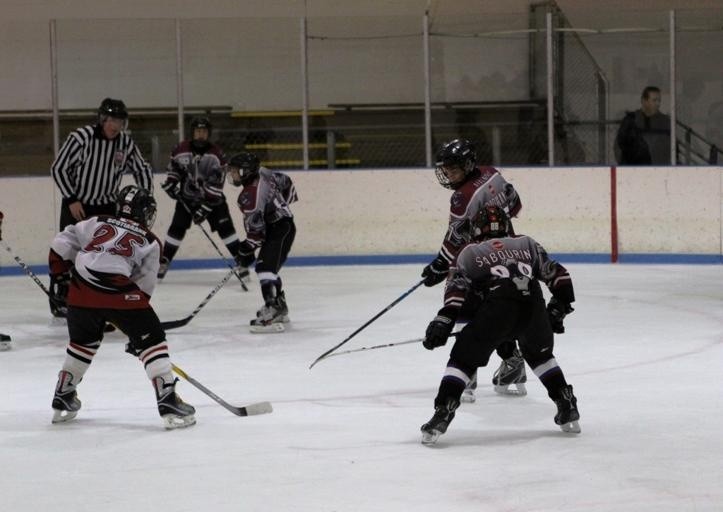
[171,364,272,415]
[160,266,242,331]
[309,273,431,371]
[315,332,459,364]
[172,193,248,290]
[1,236,115,332]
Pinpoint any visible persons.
[47,186,197,418]
[0,212,11,343]
[613,85,678,164]
[158,116,249,278]
[421,136,527,389]
[229,152,299,326]
[48,96,153,316]
[421,205,580,433]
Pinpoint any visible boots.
[420,397,460,434]
[250,290,288,325]
[239,267,249,278]
[554,385,579,425]
[52,370,81,412]
[152,376,195,416]
[492,347,527,384]
[157,256,170,278]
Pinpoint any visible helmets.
[227,153,259,186]
[434,138,481,191]
[97,98,129,133]
[116,185,157,230]
[189,115,212,139]
[468,204,510,243]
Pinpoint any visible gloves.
[161,179,182,200]
[421,255,449,286]
[422,310,454,350]
[193,202,213,224]
[234,240,256,266]
[547,295,575,333]
